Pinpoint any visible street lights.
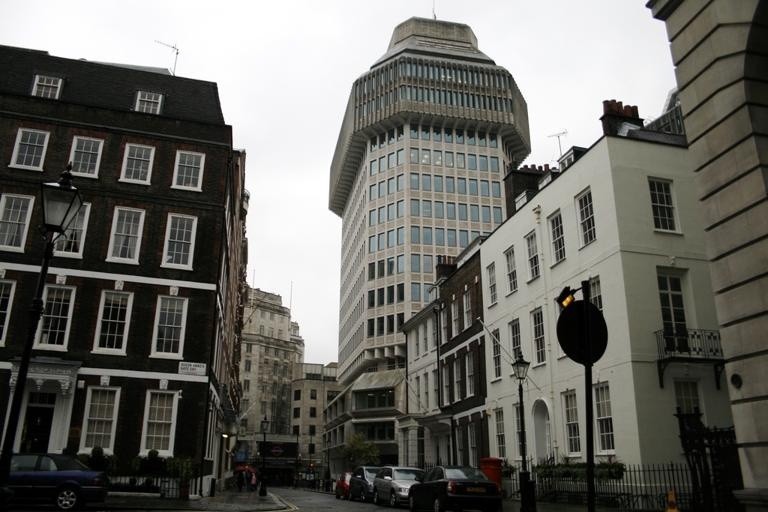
[258,413,270,498]
[0,159,86,490]
[510,351,538,512]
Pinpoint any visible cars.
[0,448,110,510]
[334,464,502,512]
